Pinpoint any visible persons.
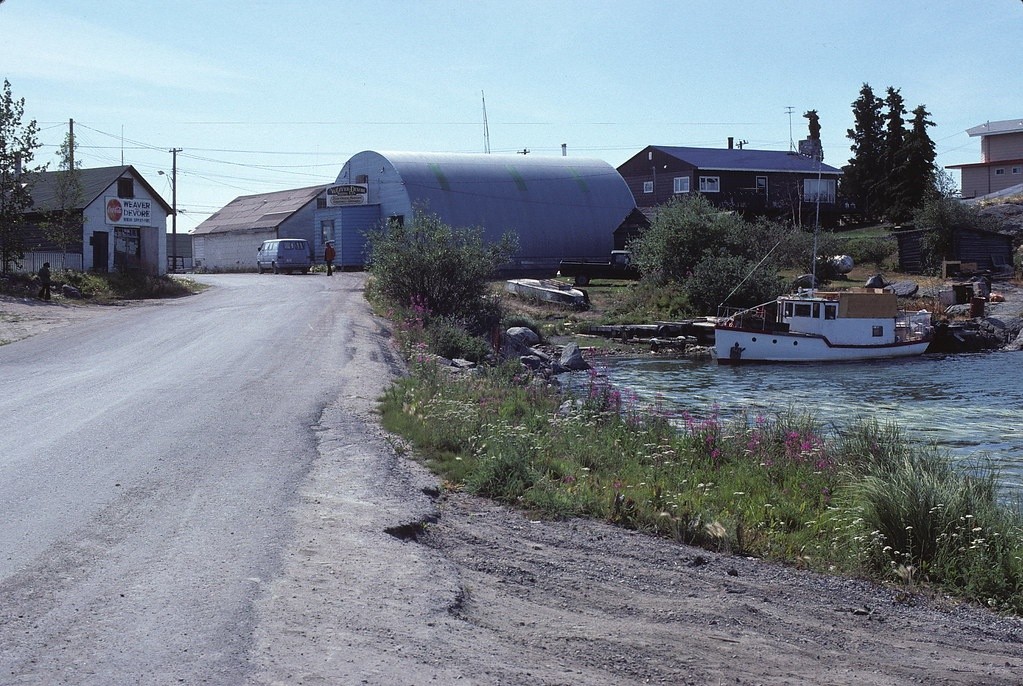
[323,242,335,276]
[38,262,51,301]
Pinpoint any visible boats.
[713,147,933,364]
[504,274,592,311]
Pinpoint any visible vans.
[256,238,312,275]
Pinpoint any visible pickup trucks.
[559,248,648,285]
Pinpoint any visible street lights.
[155,146,185,274]
